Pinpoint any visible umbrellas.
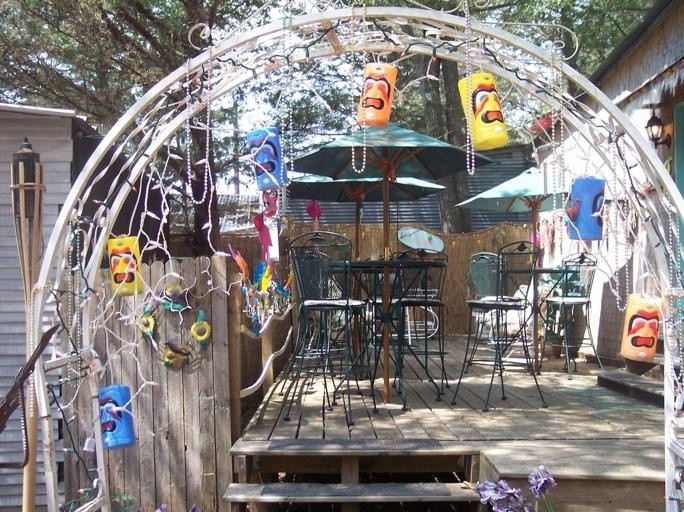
[452,163,578,374]
[274,119,491,403]
[287,170,447,359]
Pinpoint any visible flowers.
[474,466,560,511]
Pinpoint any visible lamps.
[642,103,672,152]
